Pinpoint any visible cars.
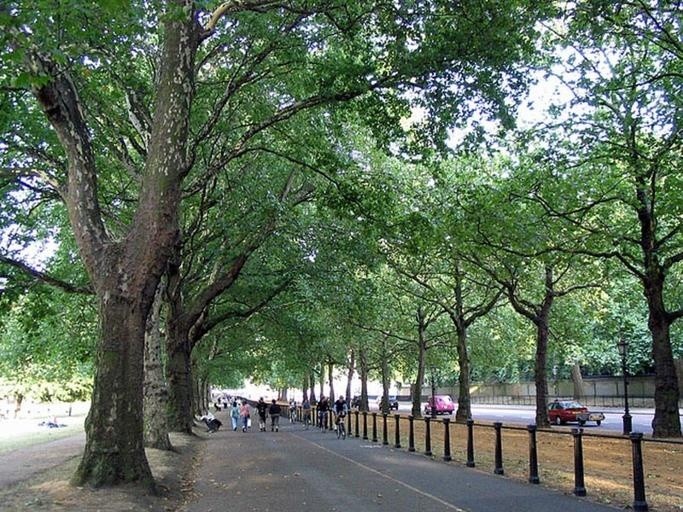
[352,395,368,409]
[425,394,455,415]
[378,396,399,410]
[547,400,588,425]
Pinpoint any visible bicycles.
[334,417,347,440]
[302,413,312,430]
[288,408,297,425]
[319,410,328,433]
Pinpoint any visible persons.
[269,399,281,432]
[231,402,239,431]
[240,402,249,432]
[289,398,296,423]
[317,396,329,429]
[303,400,311,428]
[333,396,348,417]
[254,397,267,431]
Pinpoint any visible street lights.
[617,337,632,436]
[430,364,436,418]
